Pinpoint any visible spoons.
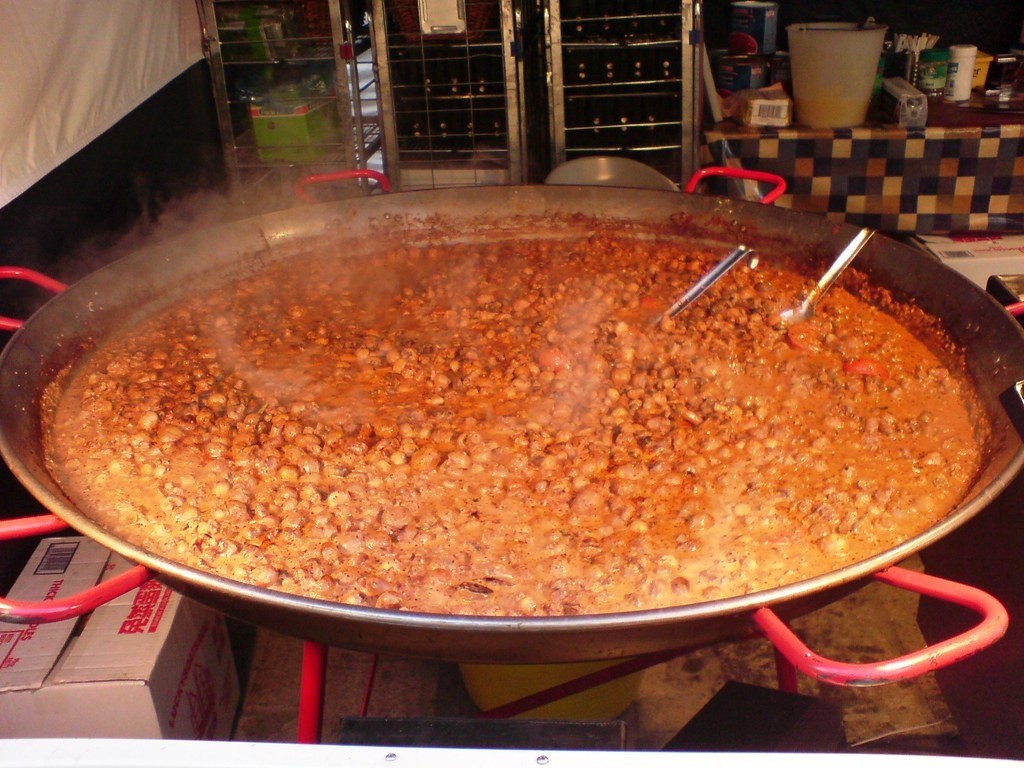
[779,224,876,325]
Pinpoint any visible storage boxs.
[0,537,241,741]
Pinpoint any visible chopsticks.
[893,32,940,55]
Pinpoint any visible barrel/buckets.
[786,22,888,127]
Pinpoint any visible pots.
[0,167,1024,687]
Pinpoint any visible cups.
[890,51,915,85]
